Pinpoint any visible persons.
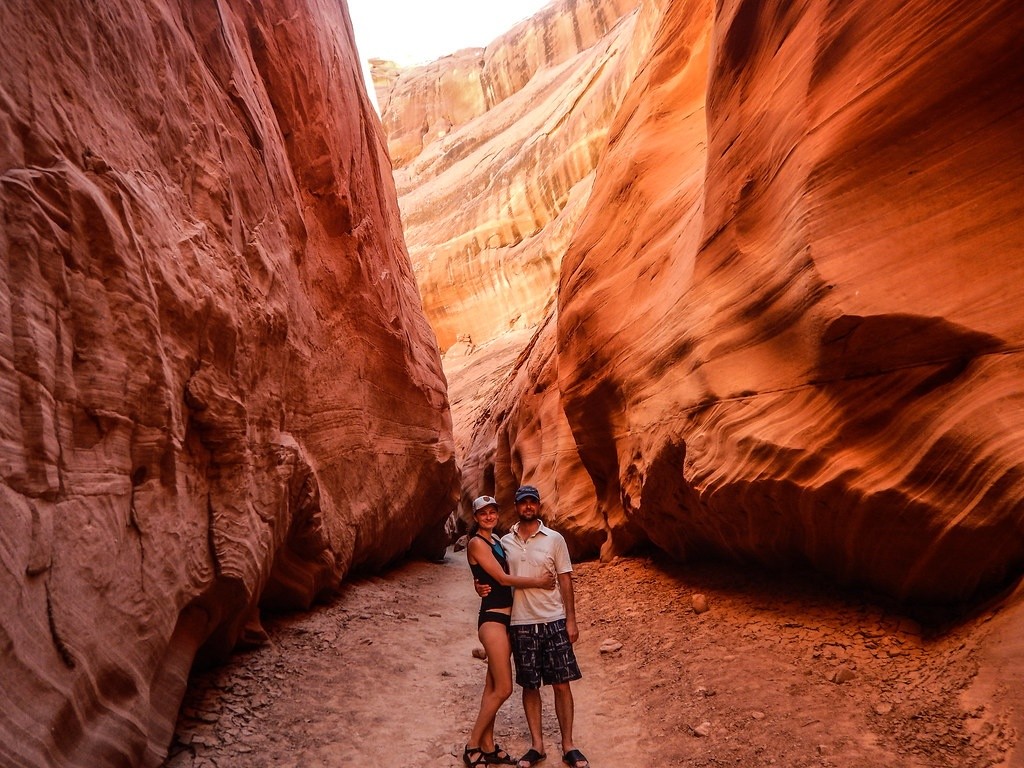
[462,496,556,768]
[475,486,591,768]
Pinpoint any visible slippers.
[562,750,590,768]
[517,749,547,768]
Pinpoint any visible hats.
[515,486,540,502]
[472,496,500,514]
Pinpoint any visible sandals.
[485,744,517,765]
[462,744,491,768]
